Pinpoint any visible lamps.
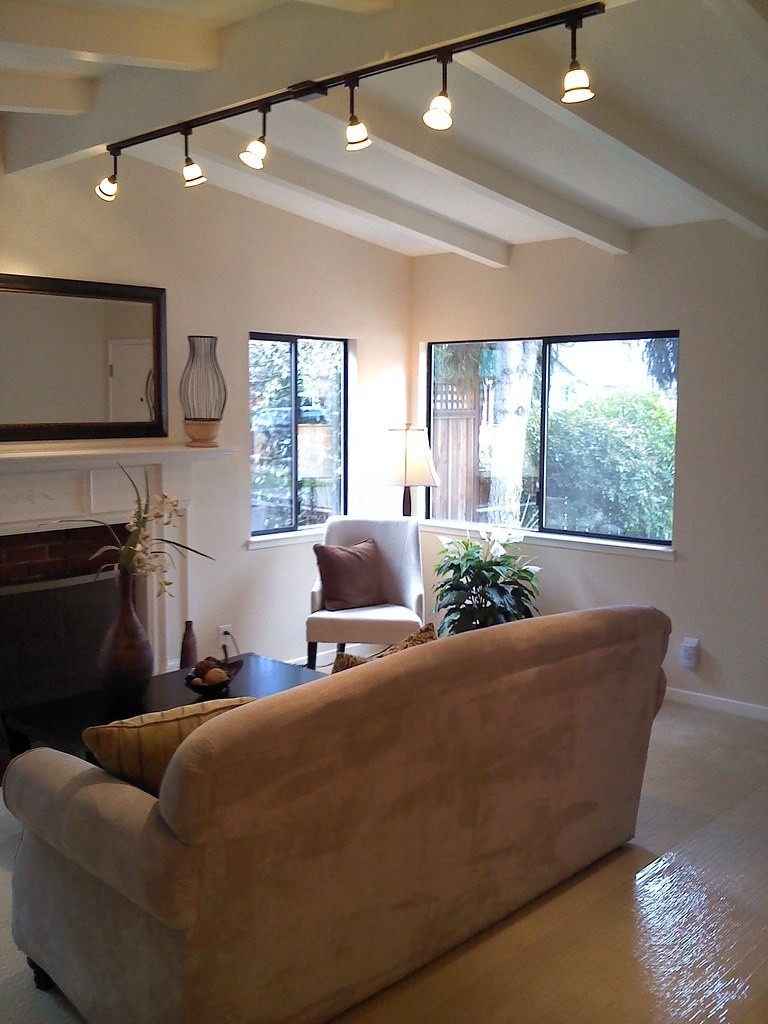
[561,17,595,104]
[180,126,207,187]
[95,146,122,202]
[385,422,441,517]
[423,53,453,130]
[238,103,271,170]
[344,78,373,152]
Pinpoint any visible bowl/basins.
[183,676,234,696]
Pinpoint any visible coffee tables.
[0,652,330,763]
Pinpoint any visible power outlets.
[217,624,232,649]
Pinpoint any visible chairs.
[304,515,426,669]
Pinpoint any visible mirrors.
[0,273,168,441]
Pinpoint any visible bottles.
[180,620,198,670]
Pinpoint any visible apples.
[206,668,227,685]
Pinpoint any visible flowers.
[38,459,215,598]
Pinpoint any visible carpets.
[0,700,767,1024]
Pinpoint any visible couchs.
[3,605,674,1024]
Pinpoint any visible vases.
[100,569,154,693]
[180,621,199,669]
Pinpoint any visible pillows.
[331,650,371,676]
[82,697,260,789]
[312,537,382,610]
[367,623,437,658]
